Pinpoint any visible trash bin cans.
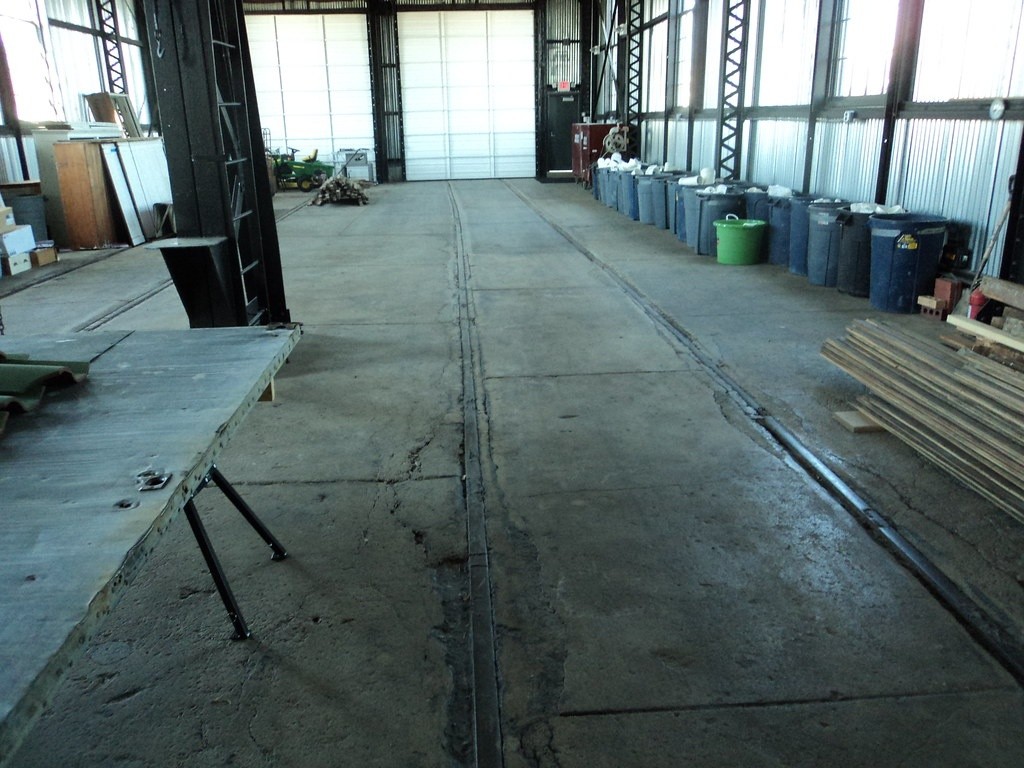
[592,160,949,315]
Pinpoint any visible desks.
[571,124,636,188]
[0,322,303,768]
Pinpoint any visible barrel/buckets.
[591,150,948,313]
[558,81,570,92]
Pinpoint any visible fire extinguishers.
[966,277,989,321]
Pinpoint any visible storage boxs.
[0,253,31,276]
[30,246,58,268]
[341,163,373,182]
[345,152,367,165]
[0,224,36,257]
[0,207,17,235]
